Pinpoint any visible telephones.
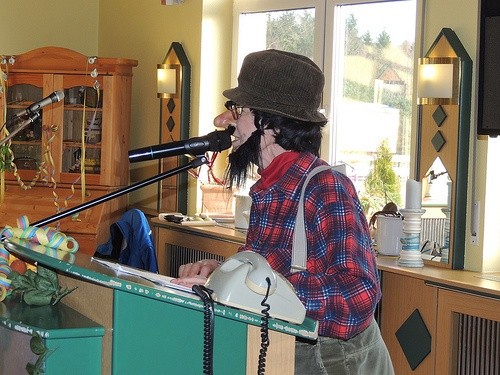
[204,250,306,325]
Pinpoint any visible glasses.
[230,104,254,120]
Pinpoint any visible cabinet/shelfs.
[152,219,500,375]
[0,47,138,257]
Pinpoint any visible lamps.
[417,57,459,105]
[157,64,180,98]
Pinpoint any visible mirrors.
[421,157,452,264]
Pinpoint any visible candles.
[406,179,422,209]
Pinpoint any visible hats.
[223,49,327,123]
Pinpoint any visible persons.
[171,49,394,375]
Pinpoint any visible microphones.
[12,90,64,119]
[128,129,232,163]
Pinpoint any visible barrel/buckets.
[232,192,252,229]
